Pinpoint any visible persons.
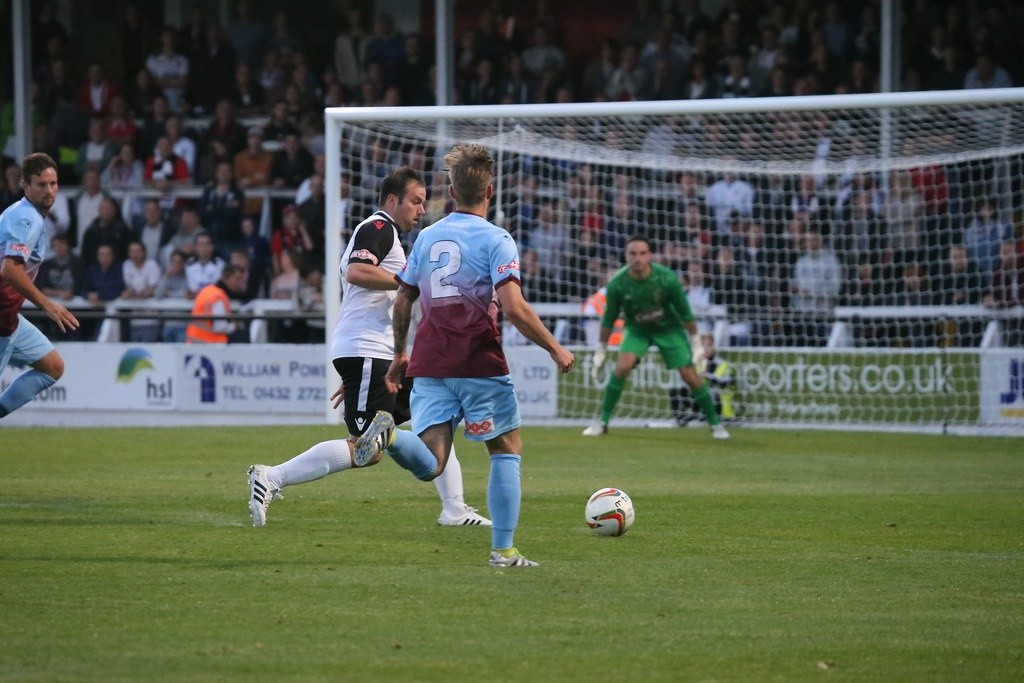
[670,332,734,426]
[246,169,493,526]
[0,153,80,418]
[582,234,730,438]
[352,146,575,566]
[1,1,1024,349]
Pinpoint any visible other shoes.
[712,424,729,439]
[584,424,606,435]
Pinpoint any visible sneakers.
[438,504,492,525]
[246,464,283,526]
[489,551,540,568]
[354,411,396,466]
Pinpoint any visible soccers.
[585,487,635,537]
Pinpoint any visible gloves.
[692,335,704,359]
[593,346,607,368]
